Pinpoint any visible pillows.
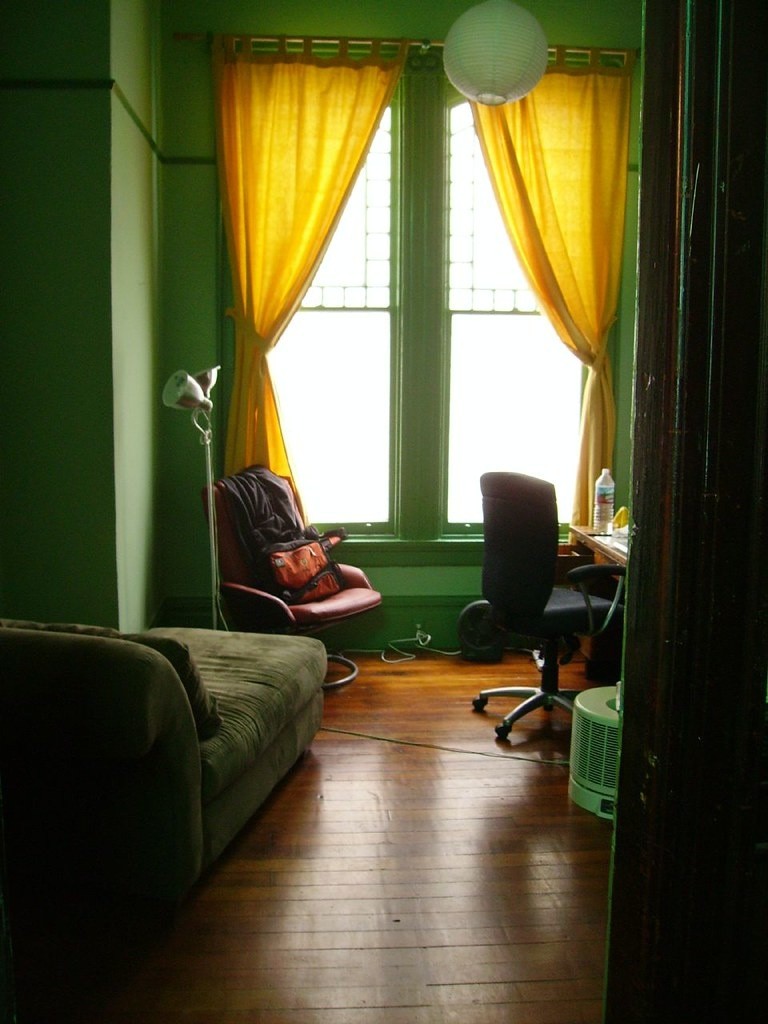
[1,619,223,740]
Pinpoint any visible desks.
[570,524,629,663]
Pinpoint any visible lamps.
[444,1,549,106]
[164,363,223,630]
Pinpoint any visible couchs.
[0,617,327,909]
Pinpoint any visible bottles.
[593,468,615,536]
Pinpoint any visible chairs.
[458,470,626,742]
[204,471,382,686]
